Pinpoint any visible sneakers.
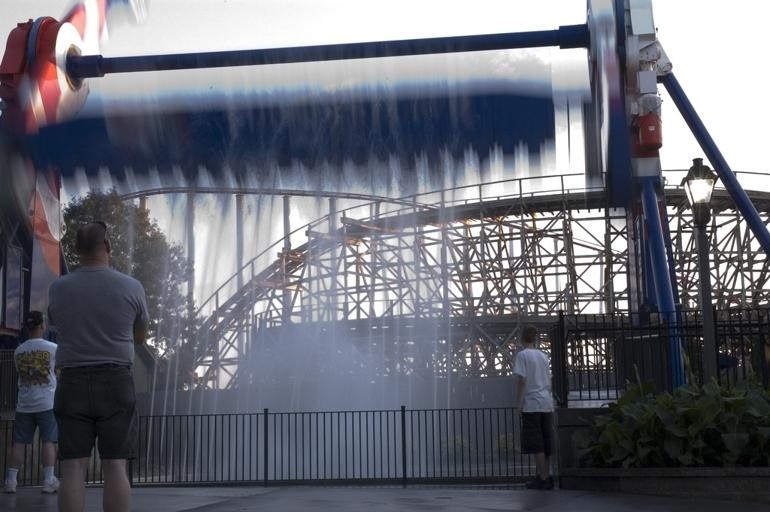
[527,480,552,489]
[42,475,60,494]
[3,479,18,493]
[525,475,554,486]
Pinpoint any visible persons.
[2,310,62,494]
[44,220,150,512]
[512,327,556,490]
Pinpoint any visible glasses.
[89,219,109,242]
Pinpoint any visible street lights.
[678,154,724,469]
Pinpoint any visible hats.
[22,310,43,329]
